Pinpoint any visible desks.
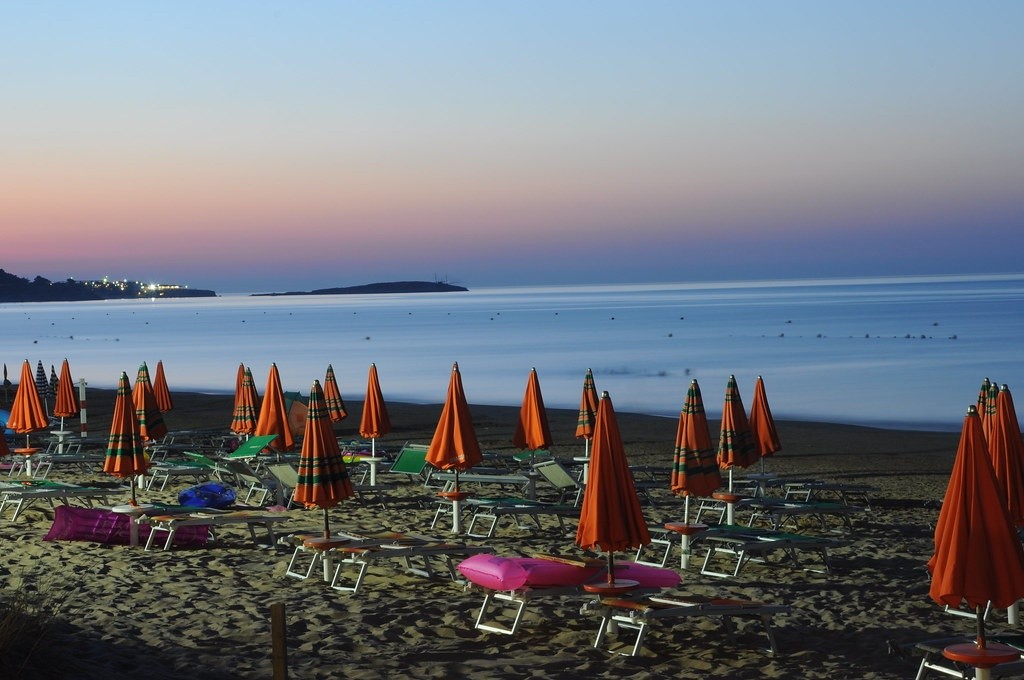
[665,522,708,570]
[747,473,777,498]
[14,448,43,476]
[112,503,154,546]
[517,472,542,497]
[573,456,592,485]
[138,463,157,489]
[302,537,352,583]
[360,457,388,486]
[436,491,476,533]
[143,441,155,450]
[583,579,640,633]
[943,643,1021,680]
[50,431,74,455]
[712,492,748,526]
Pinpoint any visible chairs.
[0,426,1024,680]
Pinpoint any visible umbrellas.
[929,405,1024,650]
[255,363,294,463]
[359,363,393,458]
[512,368,553,472]
[671,379,720,523]
[230,363,261,442]
[425,363,483,491]
[104,374,144,507]
[717,374,757,494]
[7,359,50,448]
[576,369,599,458]
[36,358,79,432]
[750,376,782,473]
[294,380,355,537]
[976,378,1024,528]
[575,392,651,583]
[323,364,348,423]
[132,361,173,442]
